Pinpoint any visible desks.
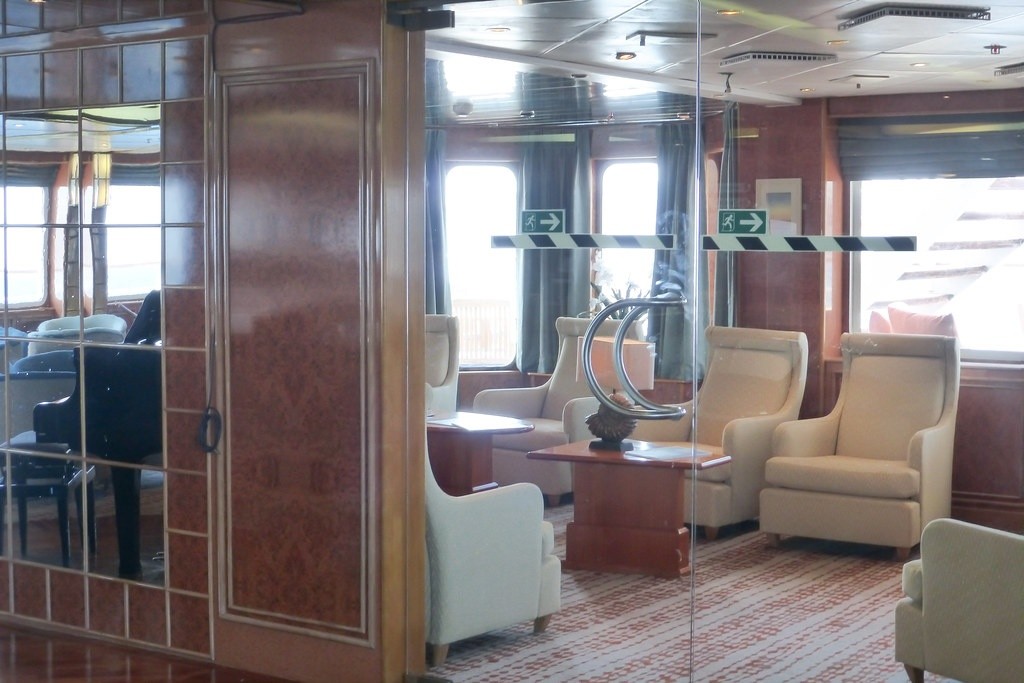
[524,435,733,578]
[423,411,536,497]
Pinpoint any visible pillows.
[887,304,961,344]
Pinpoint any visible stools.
[0,429,101,562]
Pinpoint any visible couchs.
[890,517,1023,682]
[471,315,647,507]
[424,313,464,415]
[757,331,962,563]
[625,325,810,540]
[424,414,562,667]
